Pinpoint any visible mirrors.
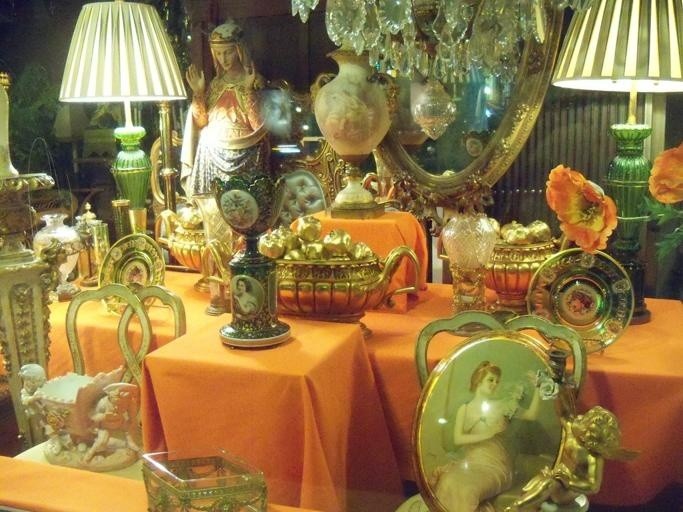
[365,2,564,239]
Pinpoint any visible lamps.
[551,0,683,326]
[58,0,190,235]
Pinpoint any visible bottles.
[32,203,111,301]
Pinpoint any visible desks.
[0,267,682,512]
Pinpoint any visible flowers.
[544,145,683,265]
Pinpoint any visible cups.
[191,192,241,317]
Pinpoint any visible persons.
[229,277,259,317]
[184,23,269,193]
[434,361,543,512]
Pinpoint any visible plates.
[98,233,165,317]
[527,248,635,354]
